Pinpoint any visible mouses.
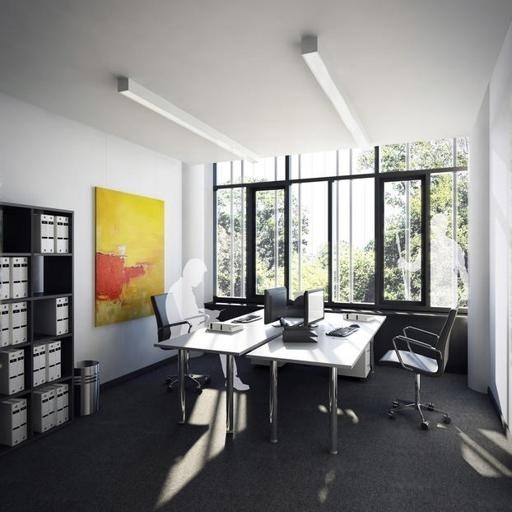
[349,324,360,328]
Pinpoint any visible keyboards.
[232,315,263,323]
[326,327,359,337]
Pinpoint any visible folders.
[209,323,245,333]
[344,313,377,323]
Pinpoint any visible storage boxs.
[10,257,29,299]
[44,383,68,426]
[34,213,53,252]
[0,258,9,300]
[33,342,46,386]
[10,303,28,344]
[0,349,24,396]
[46,340,62,382]
[0,305,11,348]
[31,387,56,434]
[34,298,68,335]
[54,217,69,253]
[1,397,27,444]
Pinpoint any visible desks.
[245,311,385,455]
[154,308,305,434]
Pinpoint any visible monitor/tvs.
[302,290,325,329]
[265,287,289,327]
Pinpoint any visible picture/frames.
[94,187,166,326]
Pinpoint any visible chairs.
[380,307,458,429]
[151,294,212,394]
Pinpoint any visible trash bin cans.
[74,360,101,416]
